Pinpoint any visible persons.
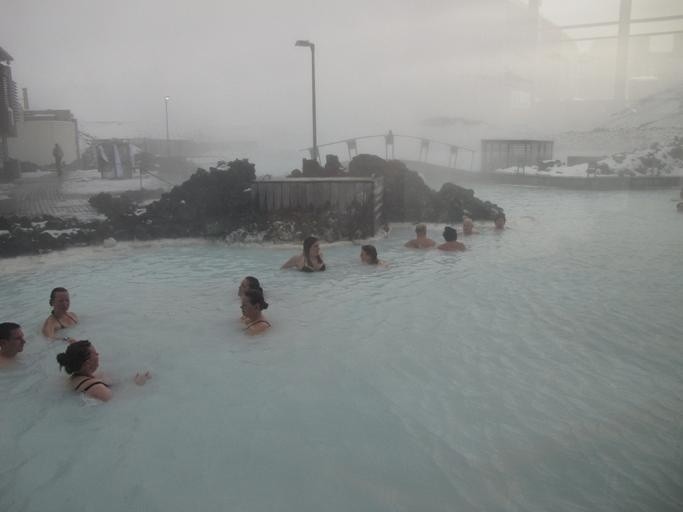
[242,289,271,336]
[490,214,509,231]
[42,287,78,343]
[279,235,326,272]
[53,144,64,173]
[0,321,27,371]
[360,245,384,267]
[237,277,263,323]
[57,340,153,402]
[436,227,465,251]
[404,224,436,248]
[456,218,483,235]
[96,146,109,177]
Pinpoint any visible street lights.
[163,97,169,146]
[296,40,317,160]
